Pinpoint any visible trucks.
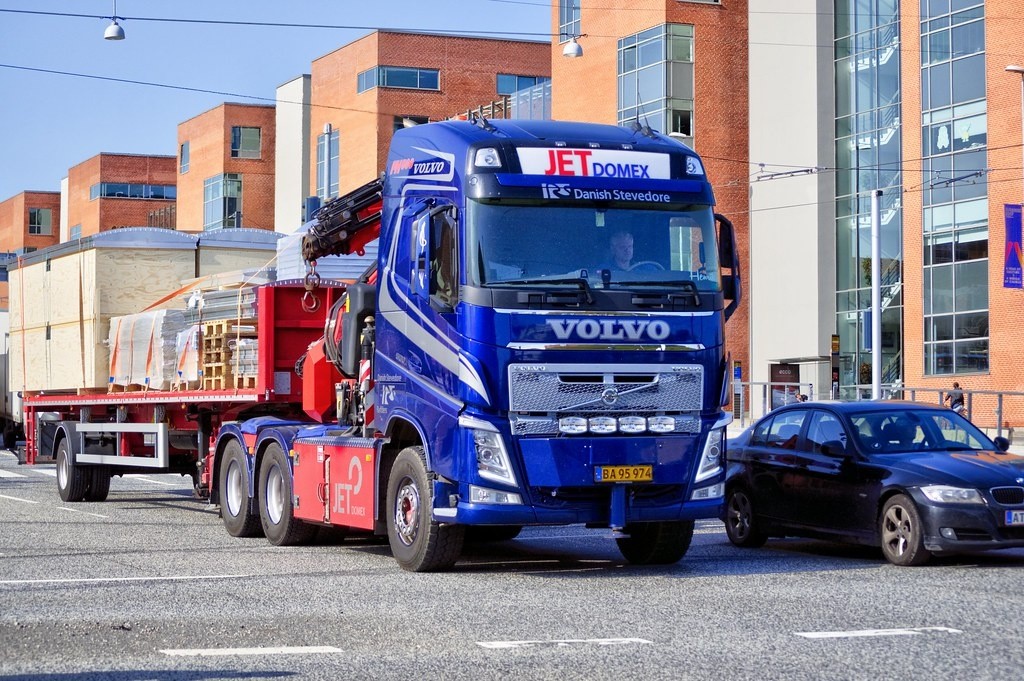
[0,309,23,450]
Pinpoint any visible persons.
[884,416,926,451]
[596,230,653,272]
[943,382,966,430]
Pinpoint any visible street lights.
[1006,66,1024,120]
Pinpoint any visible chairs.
[881,423,899,449]
[776,424,800,445]
[812,420,859,455]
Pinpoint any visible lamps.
[105,17,126,40]
[563,40,584,58]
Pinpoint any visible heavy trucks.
[19,116,741,573]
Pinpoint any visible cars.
[724,401,1024,567]
